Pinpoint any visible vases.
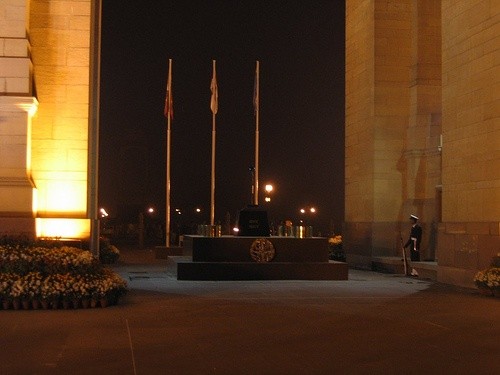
[1,298,111,310]
[478,286,500,300]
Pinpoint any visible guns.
[399,231,408,276]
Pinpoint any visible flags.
[211,65,218,114]
[164,67,173,120]
[253,62,259,109]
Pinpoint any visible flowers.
[1,272,127,300]
[474,269,500,291]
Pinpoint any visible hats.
[409,215,419,220]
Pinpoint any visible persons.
[403,214,423,276]
